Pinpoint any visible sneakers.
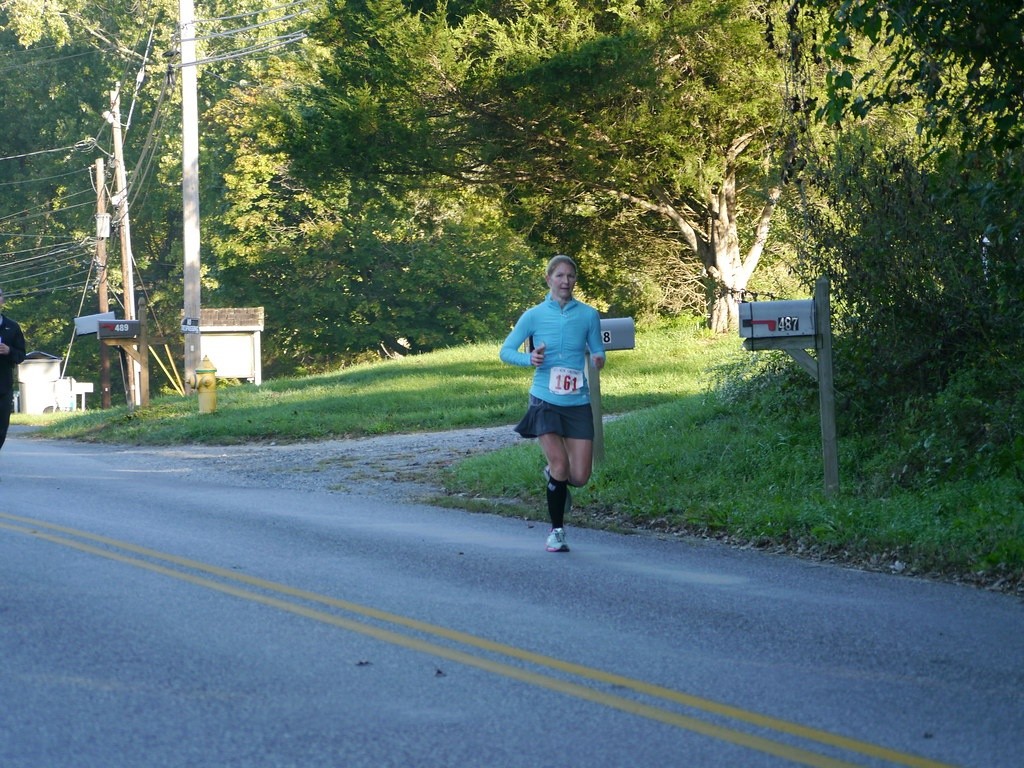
[544,528,570,552]
[544,466,572,513]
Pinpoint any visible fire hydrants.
[184,353,218,415]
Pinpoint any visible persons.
[0,288,26,449]
[500,255,605,552]
